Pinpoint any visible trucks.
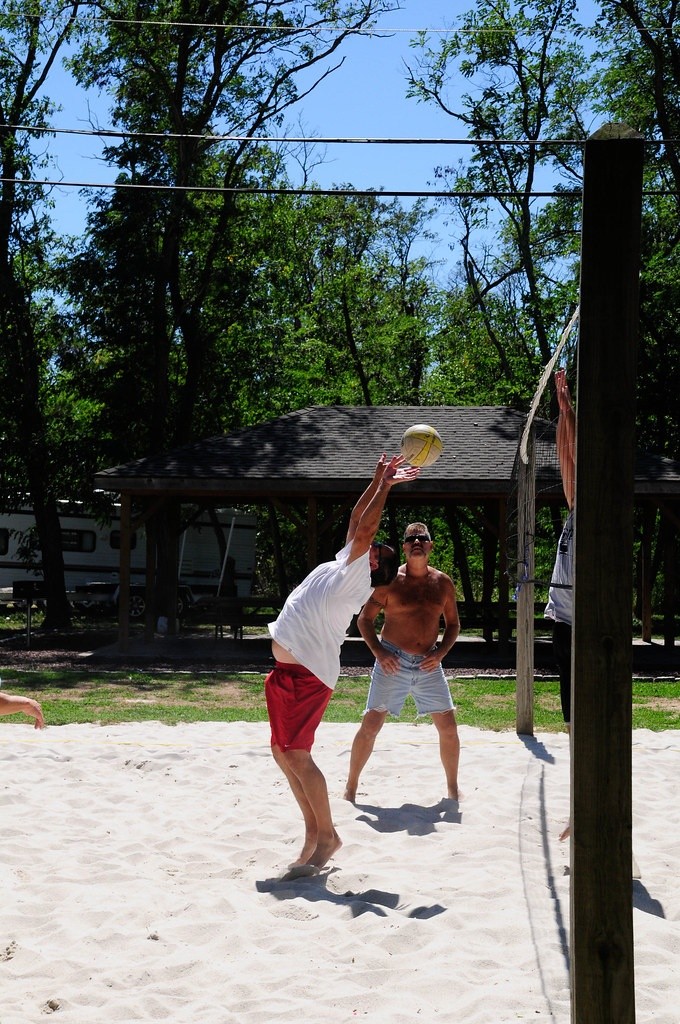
[1,492,259,624]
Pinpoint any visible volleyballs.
[399,423,444,468]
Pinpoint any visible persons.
[541,368,576,839]
[262,450,421,872]
[343,520,462,803]
[0,691,47,731]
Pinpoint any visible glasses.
[403,534,431,544]
[372,539,383,563]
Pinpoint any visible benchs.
[178,600,549,642]
